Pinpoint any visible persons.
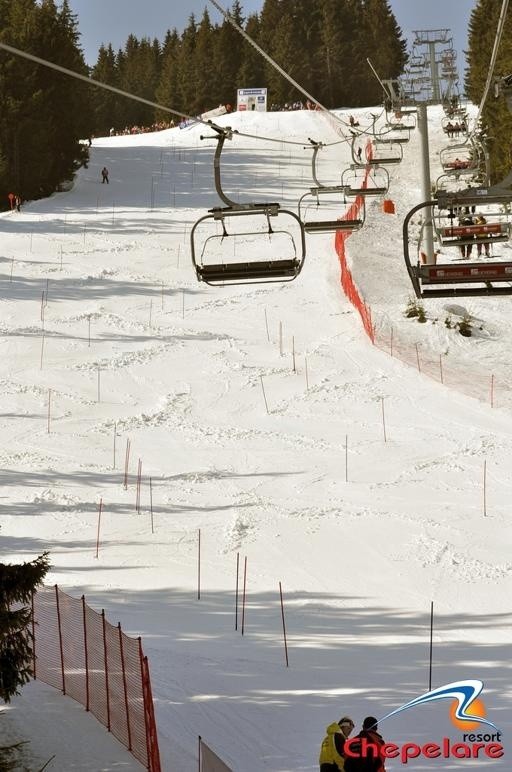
[453,157,463,179]
[316,716,356,771]
[107,117,188,137]
[445,93,467,140]
[356,145,363,161]
[349,114,356,127]
[458,215,475,258]
[14,194,21,213]
[473,211,492,258]
[101,166,109,184]
[267,99,320,112]
[453,187,464,216]
[464,183,477,214]
[341,716,388,771]
[226,102,233,114]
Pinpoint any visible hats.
[363,717,377,730]
[338,718,355,727]
